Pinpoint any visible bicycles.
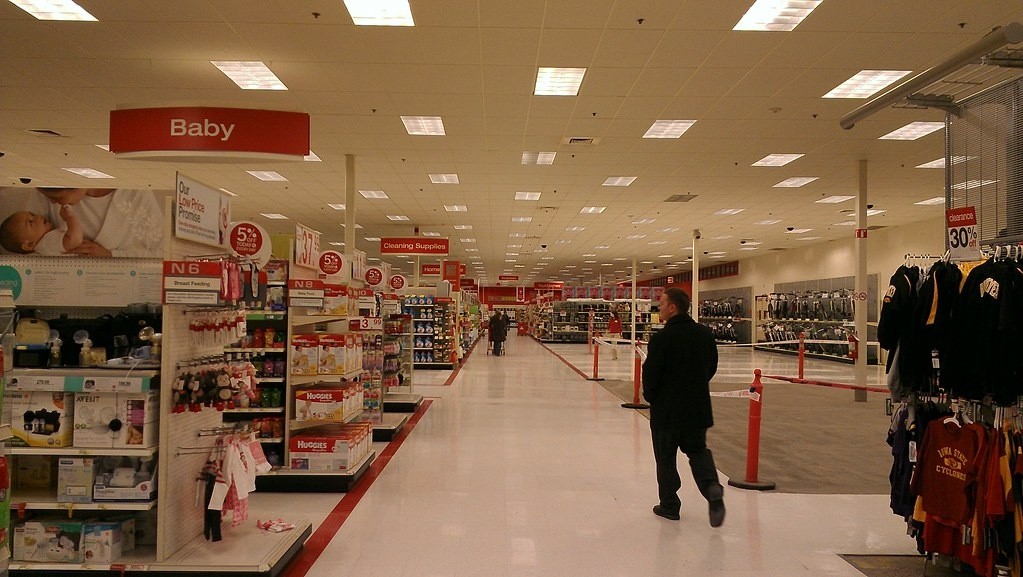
[697,296,744,345]
[755,287,854,358]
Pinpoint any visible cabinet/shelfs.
[0,196,312,577]
[223,235,375,494]
[0,290,15,577]
[324,287,485,442]
[493,305,661,343]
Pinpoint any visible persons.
[292,345,304,367]
[641,286,727,528]
[126,425,143,445]
[320,345,329,366]
[488,310,509,356]
[83,459,91,466]
[607,310,623,361]
[0,187,165,259]
[299,400,311,420]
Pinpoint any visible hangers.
[943,399,981,427]
[908,390,933,411]
[993,245,1023,262]
[904,250,954,282]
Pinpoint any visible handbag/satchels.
[508,328,510,331]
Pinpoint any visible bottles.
[252,416,282,437]
[253,328,276,349]
[413,322,433,333]
[385,357,404,373]
[383,321,402,334]
[268,452,280,466]
[414,351,433,362]
[250,387,281,407]
[405,293,433,305]
[413,337,432,348]
[382,374,399,387]
[383,338,402,355]
[226,350,285,377]
[403,308,433,319]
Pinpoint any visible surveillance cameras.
[596,205,874,286]
[542,245,547,248]
[19,178,32,184]
[0,153,4,157]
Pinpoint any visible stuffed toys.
[171,367,237,415]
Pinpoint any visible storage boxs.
[289,422,373,472]
[271,286,287,311]
[6,456,159,504]
[13,517,136,563]
[291,331,363,376]
[12,390,74,447]
[308,284,359,317]
[73,390,159,449]
[268,261,287,285]
[295,382,364,421]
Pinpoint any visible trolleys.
[486,327,505,356]
[517,321,526,337]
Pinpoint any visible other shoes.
[653,505,680,520]
[708,482,726,527]
